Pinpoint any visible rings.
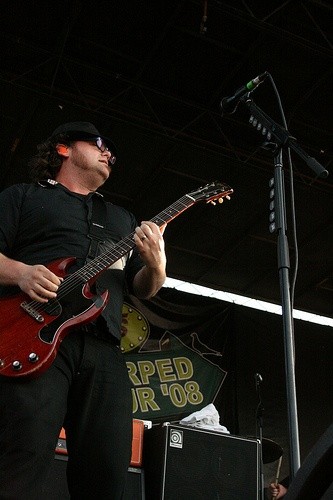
[140,235,147,241]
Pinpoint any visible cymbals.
[240,435,284,464]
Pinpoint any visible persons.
[0,122,167,500]
[269,475,291,500]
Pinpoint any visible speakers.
[282,423,333,500]
[46,454,145,500]
[143,422,261,500]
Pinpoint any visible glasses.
[65,137,116,165]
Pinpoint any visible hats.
[50,121,112,146]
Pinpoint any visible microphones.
[221,71,268,114]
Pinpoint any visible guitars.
[0,178,235,379]
